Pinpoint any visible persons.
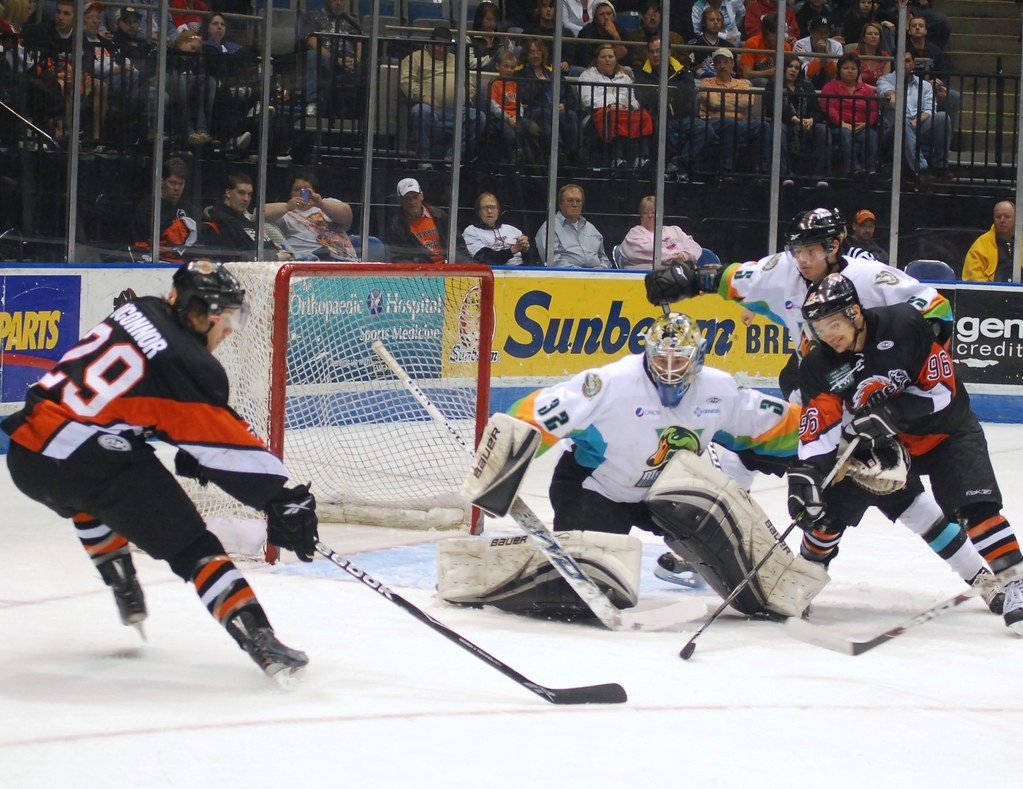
[1,1,955,188]
[0,264,318,692]
[787,271,1023,636]
[646,206,1006,618]
[460,191,542,266]
[120,157,234,263]
[534,182,614,270]
[839,210,889,265]
[254,170,362,263]
[617,195,703,270]
[383,177,476,265]
[961,199,1023,284]
[210,173,319,262]
[435,311,831,627]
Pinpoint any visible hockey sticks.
[313,535,629,705]
[368,337,712,633]
[677,434,862,661]
[783,562,1022,658]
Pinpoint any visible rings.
[296,203,299,206]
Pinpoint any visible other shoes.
[0,131,963,196]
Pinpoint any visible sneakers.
[654,551,707,590]
[227,611,310,688]
[965,563,1006,616]
[795,546,839,618]
[1003,579,1023,637]
[106,555,150,644]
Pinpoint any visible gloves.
[842,405,905,451]
[787,467,830,531]
[266,481,319,563]
[644,259,702,305]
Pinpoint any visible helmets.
[800,272,863,338]
[783,207,848,259]
[174,259,246,327]
[644,312,708,408]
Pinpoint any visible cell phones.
[300,189,309,203]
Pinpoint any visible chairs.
[92,0,956,281]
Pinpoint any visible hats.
[853,210,876,225]
[594,0,616,16]
[396,177,421,198]
[712,47,733,60]
